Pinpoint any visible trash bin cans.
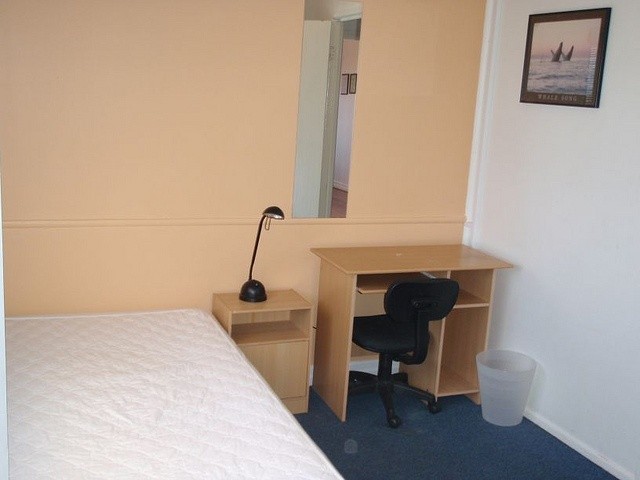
[476,349,537,426]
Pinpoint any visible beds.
[3,309,346,476]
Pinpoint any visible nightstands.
[212,289,307,415]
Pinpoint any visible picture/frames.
[520,7,611,108]
[350,74,357,94]
[340,74,348,95]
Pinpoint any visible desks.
[309,244,516,421]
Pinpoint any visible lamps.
[239,206,285,303]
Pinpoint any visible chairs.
[352,277,460,427]
